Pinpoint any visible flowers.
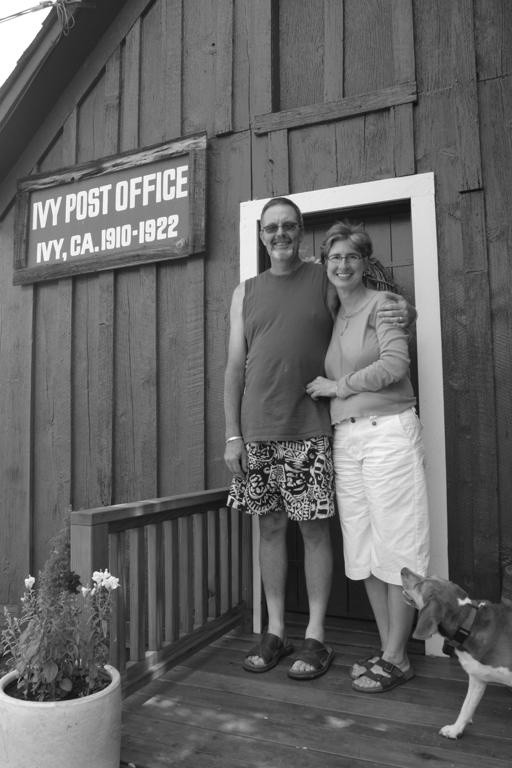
[1,567,120,700]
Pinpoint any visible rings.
[399,316,404,322]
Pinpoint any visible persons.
[220,196,417,684]
[304,219,433,694]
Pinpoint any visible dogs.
[398,565,512,742]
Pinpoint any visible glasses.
[259,221,300,233]
[327,254,363,263]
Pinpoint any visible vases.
[0,659,121,768]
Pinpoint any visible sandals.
[349,651,416,693]
[241,633,293,673]
[287,638,336,680]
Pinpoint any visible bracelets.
[223,435,243,443]
